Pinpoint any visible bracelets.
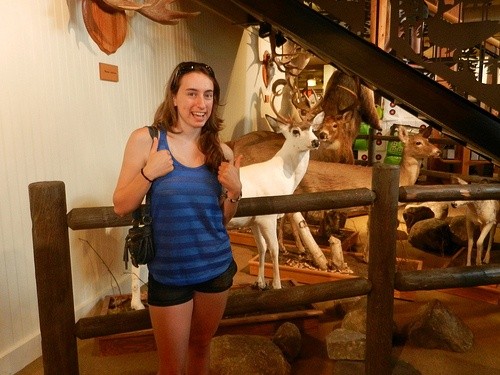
[224,189,243,203]
[141,167,154,183]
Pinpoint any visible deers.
[93,0,500,310]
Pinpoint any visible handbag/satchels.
[124,215,155,267]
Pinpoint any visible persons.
[112,61,244,375]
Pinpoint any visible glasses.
[173,62,214,86]
